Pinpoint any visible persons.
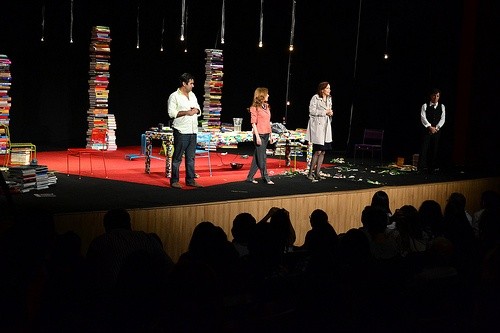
[168,72,204,188]
[419,88,445,171]
[247,88,276,184]
[307,82,333,182]
[0,191,500,333]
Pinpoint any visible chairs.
[67,128,108,179]
[1,123,36,176]
[354,123,383,163]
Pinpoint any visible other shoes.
[267,181,274,185]
[317,176,327,180]
[252,180,258,184]
[307,177,319,183]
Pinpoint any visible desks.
[160,128,313,170]
[145,131,212,178]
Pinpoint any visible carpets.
[31,147,337,189]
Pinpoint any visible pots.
[230,162,244,169]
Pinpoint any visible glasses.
[308,215,312,218]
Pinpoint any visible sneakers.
[187,182,204,187]
[170,182,185,188]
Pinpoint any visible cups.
[233,118,243,132]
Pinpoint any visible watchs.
[197,109,200,114]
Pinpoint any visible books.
[0,54,58,193]
[266,128,317,171]
[195,49,237,158]
[86,25,117,151]
